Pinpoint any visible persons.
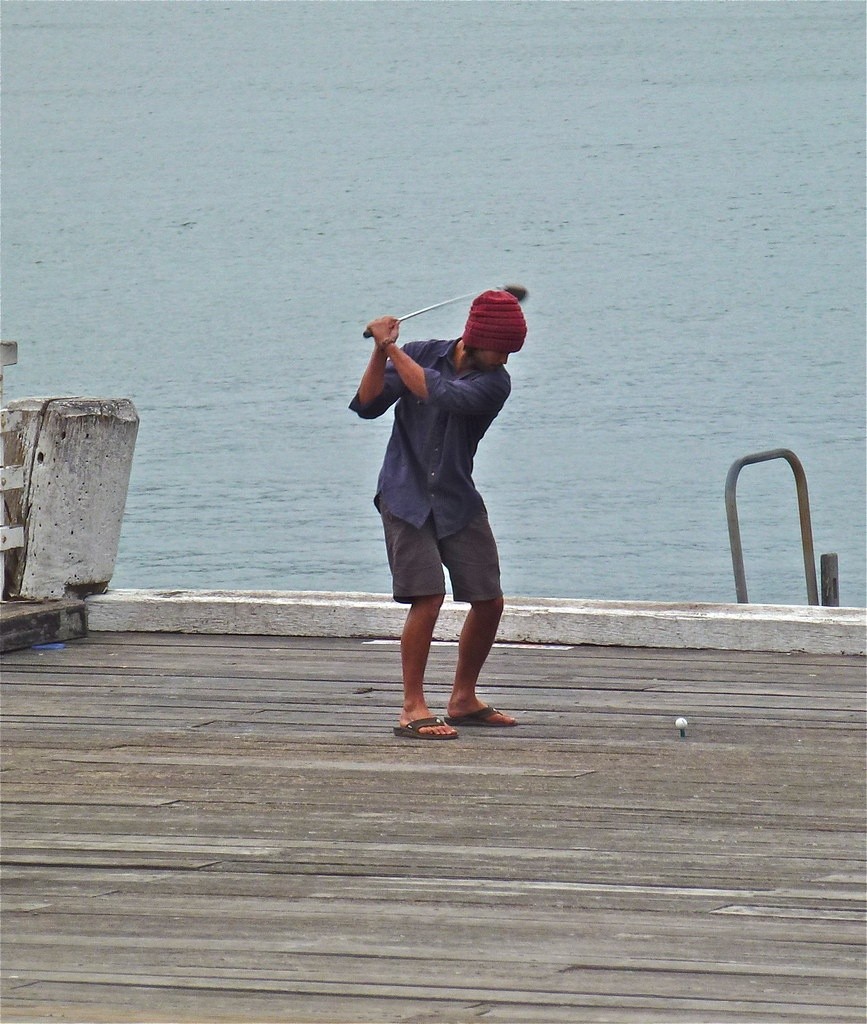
[349,290,528,740]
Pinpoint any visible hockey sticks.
[362,282,527,338]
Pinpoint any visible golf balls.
[675,717,688,730]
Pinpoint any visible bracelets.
[381,338,396,352]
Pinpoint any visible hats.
[462,289,528,353]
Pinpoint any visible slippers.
[443,704,518,727]
[392,717,459,739]
